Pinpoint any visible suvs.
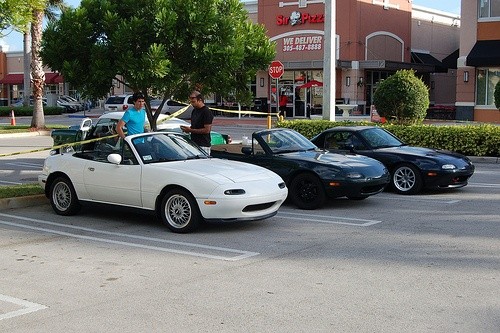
[103,94,135,111]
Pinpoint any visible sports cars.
[209,128,391,210]
[308,125,475,196]
[37,130,289,234]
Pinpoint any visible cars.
[68,110,228,155]
[10,94,92,113]
[149,99,195,119]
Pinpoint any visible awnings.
[410,52,448,74]
[442,48,459,69]
[465,40,500,67]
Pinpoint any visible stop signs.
[268,60,285,80]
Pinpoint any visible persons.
[115,93,146,150]
[180,91,213,147]
[86,97,97,112]
[279,91,288,119]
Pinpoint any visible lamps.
[464,71,469,82]
[260,77,264,87]
[360,77,363,83]
[346,76,350,86]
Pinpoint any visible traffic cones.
[11,109,16,126]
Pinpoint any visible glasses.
[188,97,196,99]
